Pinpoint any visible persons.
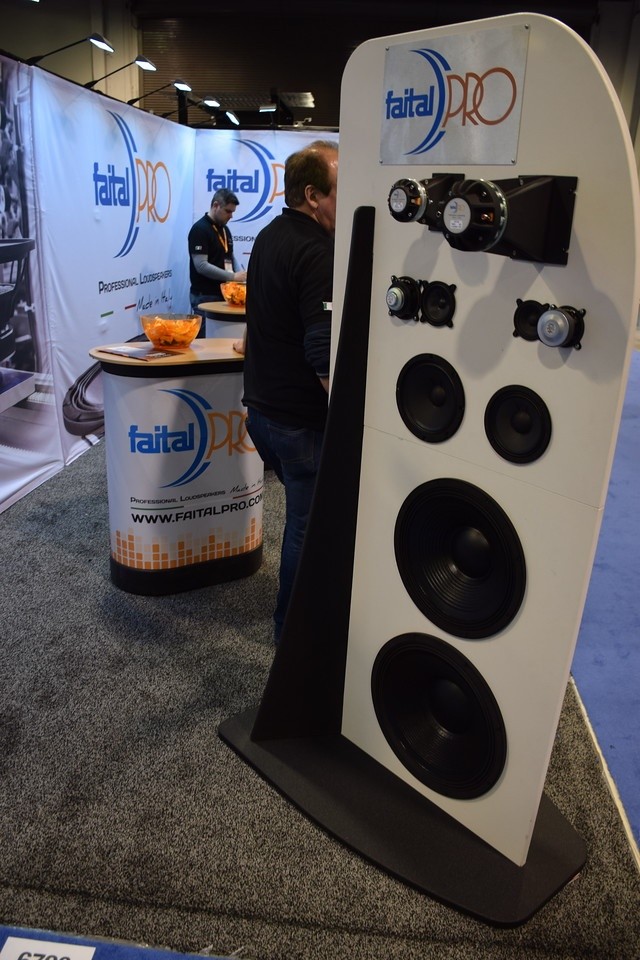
[188,188,247,339]
[241,140,337,646]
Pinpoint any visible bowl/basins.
[141,314,202,355]
[220,282,246,309]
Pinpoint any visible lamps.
[190,110,240,126]
[259,103,277,125]
[85,55,157,89]
[127,78,192,105]
[27,32,115,66]
[161,96,220,118]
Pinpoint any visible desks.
[198,302,247,338]
[89,337,263,596]
